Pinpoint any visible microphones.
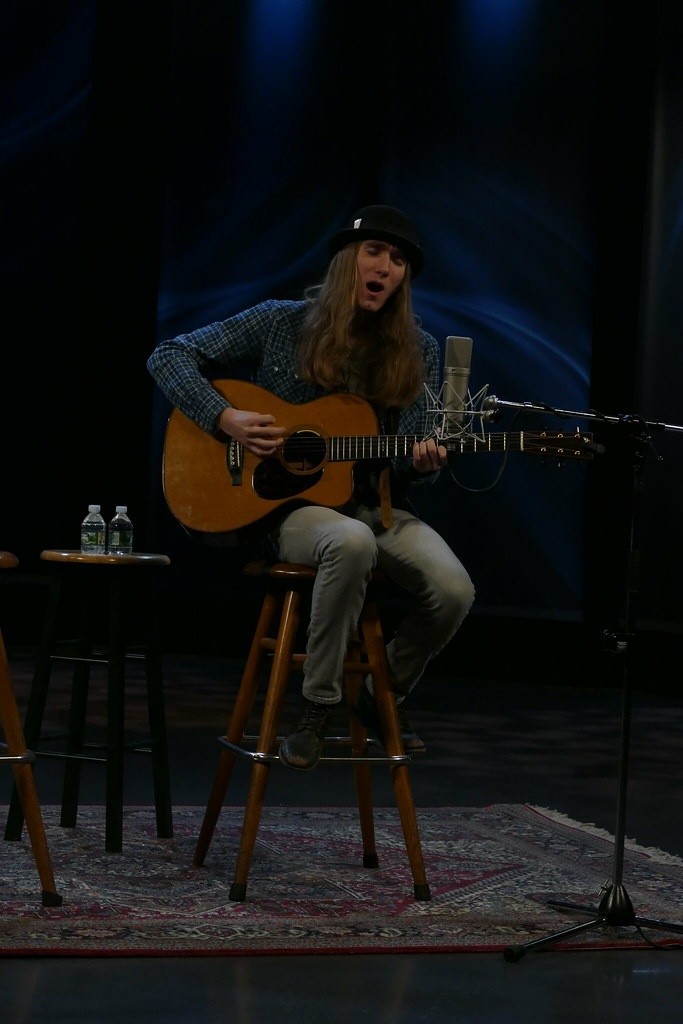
[443,335,474,431]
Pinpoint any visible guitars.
[161,381,607,547]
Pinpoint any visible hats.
[323,205,425,279]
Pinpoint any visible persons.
[144,205,476,769]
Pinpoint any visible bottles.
[107,506,133,555]
[81,504,107,555]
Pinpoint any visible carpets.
[0,799,682,958]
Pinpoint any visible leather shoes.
[350,680,427,753]
[280,698,337,772]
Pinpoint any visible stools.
[38,548,181,854]
[193,553,434,906]
[0,548,63,909]
